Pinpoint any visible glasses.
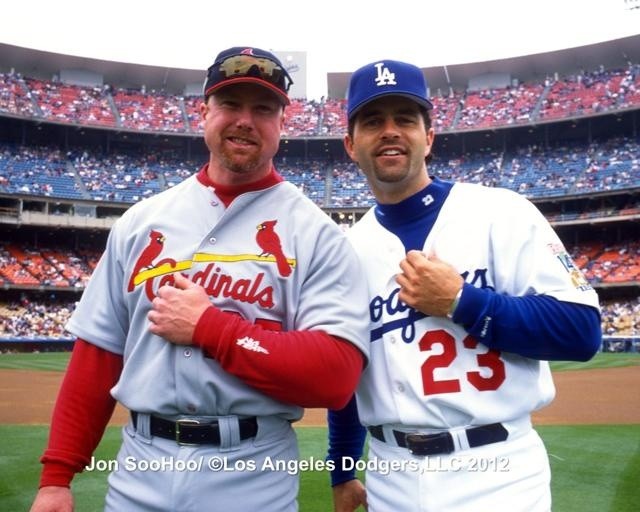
[208,54,293,93]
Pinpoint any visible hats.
[347,59,433,121]
[203,46,291,108]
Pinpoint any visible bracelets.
[446,289,466,320]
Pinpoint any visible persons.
[323,58,603,512]
[26,44,373,512]
[0,59,640,342]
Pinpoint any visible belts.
[367,423,509,457]
[129,409,260,448]
